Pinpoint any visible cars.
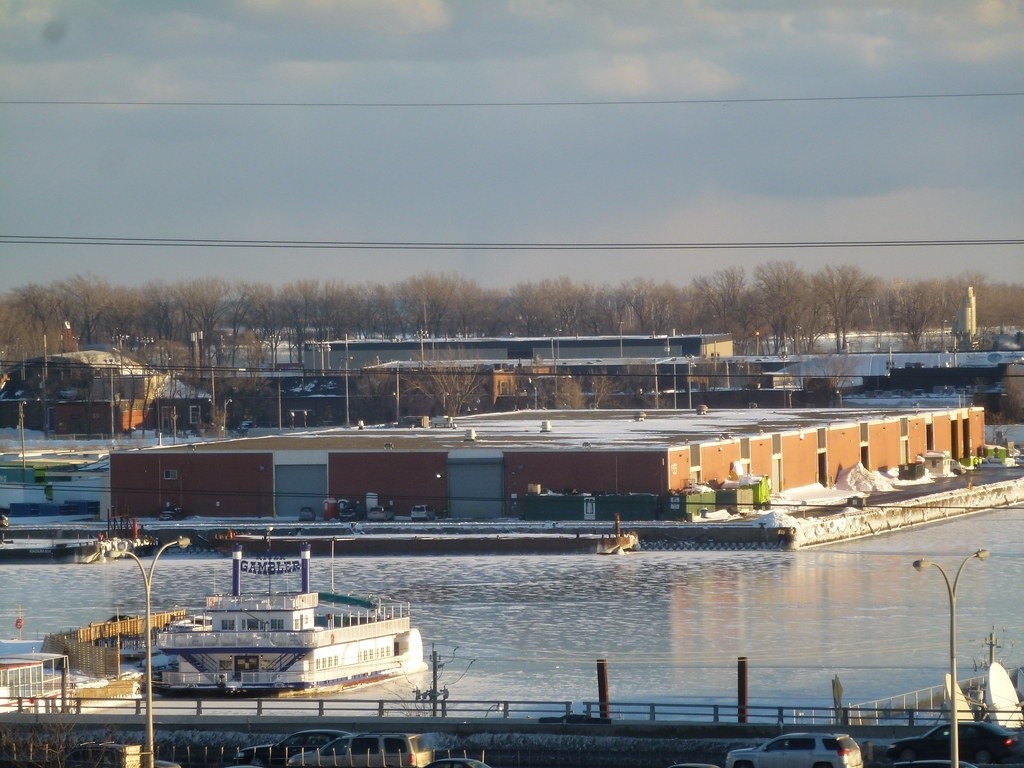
[339,506,358,523]
[234,728,355,767]
[419,757,494,767]
[159,504,184,522]
[669,762,720,768]
[298,505,316,522]
[366,506,386,522]
[888,758,977,767]
[886,720,1024,767]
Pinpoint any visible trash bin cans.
[716,479,772,516]
[324,498,337,523]
[519,488,716,522]
[898,452,949,483]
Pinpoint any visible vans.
[55,740,140,768]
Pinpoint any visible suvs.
[285,734,437,767]
[725,734,865,768]
[410,504,435,521]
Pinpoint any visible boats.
[147,540,428,699]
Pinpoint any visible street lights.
[618,321,624,358]
[102,357,120,439]
[911,548,993,768]
[415,329,429,372]
[223,398,234,439]
[755,331,759,356]
[108,533,193,768]
[778,352,787,407]
[684,353,693,408]
[553,327,563,360]
[316,340,331,378]
[20,400,26,469]
